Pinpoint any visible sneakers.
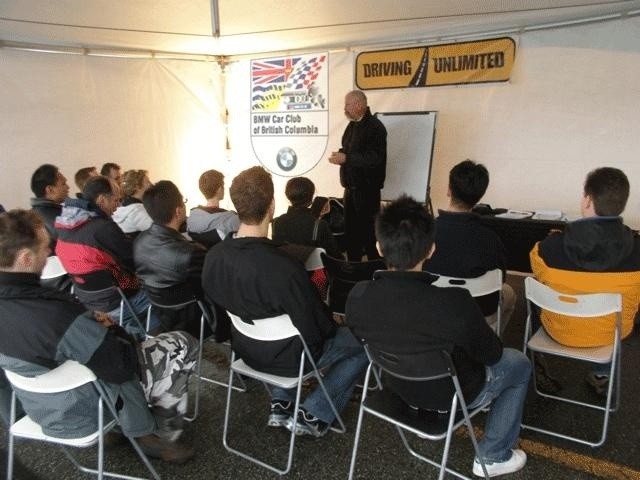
[531,367,615,403]
[266,400,294,427]
[283,409,332,440]
[137,432,196,464]
[471,447,528,479]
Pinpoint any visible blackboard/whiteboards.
[373,111,438,203]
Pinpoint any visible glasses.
[178,198,188,205]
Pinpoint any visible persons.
[329,90,387,261]
[0,161,640,478]
